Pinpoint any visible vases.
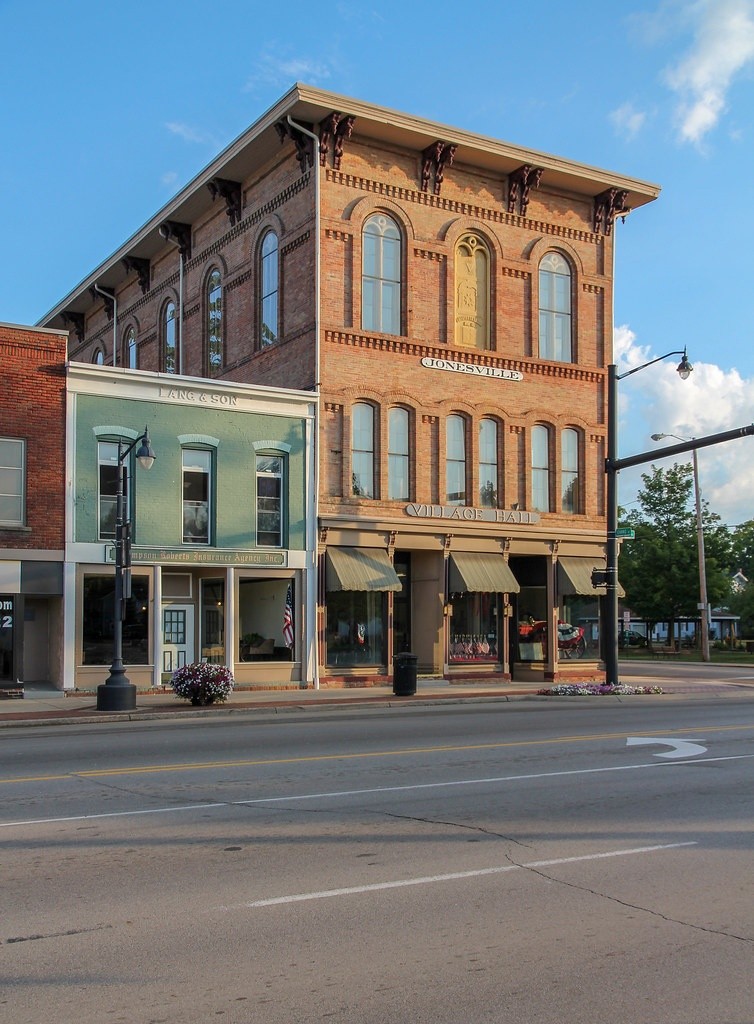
[189,695,215,706]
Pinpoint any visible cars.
[80,641,141,666]
[618,630,649,648]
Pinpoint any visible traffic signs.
[615,527,635,539]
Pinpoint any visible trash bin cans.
[391,652,419,696]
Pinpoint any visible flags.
[282,587,293,649]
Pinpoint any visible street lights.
[651,433,711,664]
[97,428,158,710]
[607,345,694,684]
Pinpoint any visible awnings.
[449,552,520,593]
[325,546,402,592]
[557,557,626,599]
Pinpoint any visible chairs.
[244,639,276,661]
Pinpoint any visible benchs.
[651,646,680,660]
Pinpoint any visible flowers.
[169,662,234,705]
